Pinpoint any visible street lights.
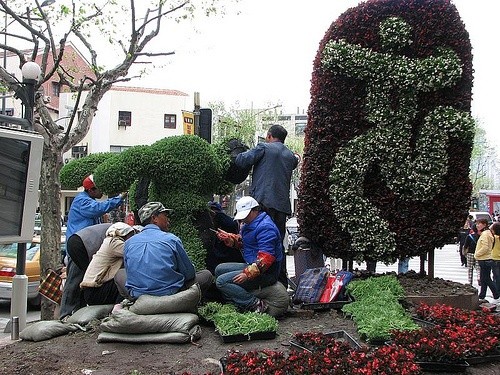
[246,104,283,125]
[22,61,41,130]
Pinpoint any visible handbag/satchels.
[222,139,253,184]
[293,264,350,304]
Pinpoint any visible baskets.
[290,276,356,309]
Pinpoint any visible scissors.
[208,226,233,241]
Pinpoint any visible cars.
[468,211,494,229]
[0,235,67,311]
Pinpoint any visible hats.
[232,195,259,221]
[138,201,173,224]
[106,222,136,238]
[82,175,97,190]
[132,225,144,232]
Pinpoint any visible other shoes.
[489,297,500,304]
[60,308,79,322]
[250,299,268,314]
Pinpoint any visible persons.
[474,218,497,304]
[59,175,127,321]
[67,222,143,305]
[213,196,283,314]
[114,201,212,300]
[235,125,300,290]
[490,223,500,304]
[457,212,500,286]
